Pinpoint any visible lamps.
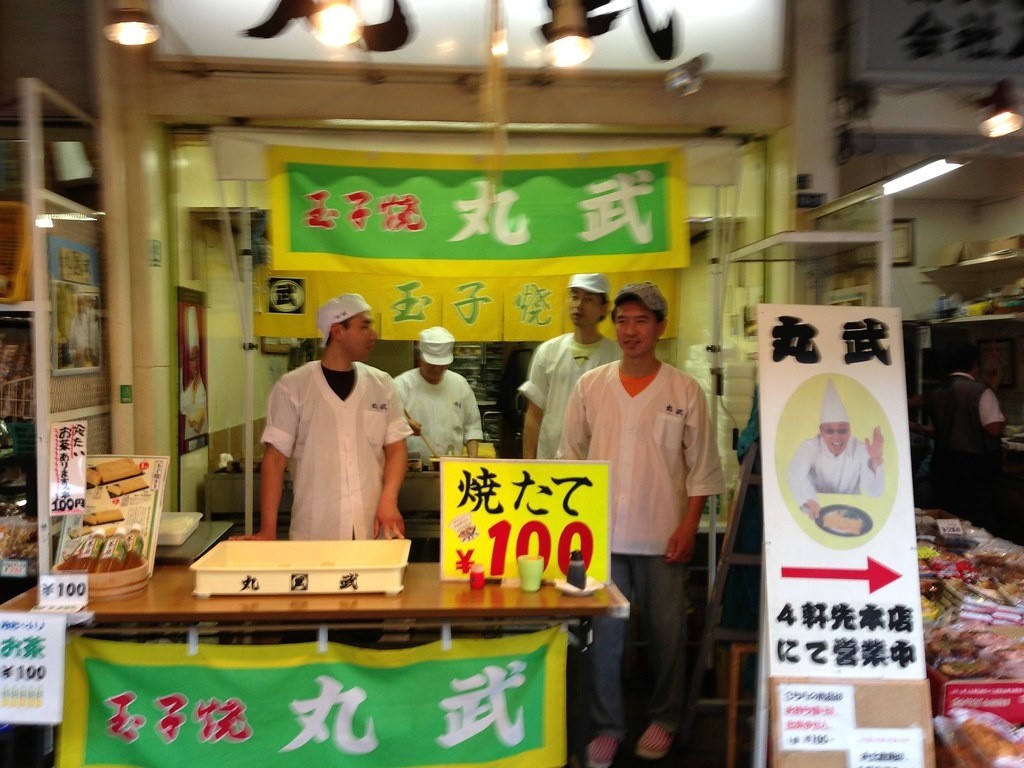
[310,0,365,50]
[102,7,162,48]
[542,0,595,69]
[883,152,972,196]
[971,77,1024,140]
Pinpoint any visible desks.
[0,562,631,768]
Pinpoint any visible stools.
[716,642,759,768]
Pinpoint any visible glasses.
[821,428,849,434]
[190,355,200,361]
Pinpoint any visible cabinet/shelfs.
[154,519,236,564]
[920,248,1024,326]
[413,341,505,448]
[0,78,111,589]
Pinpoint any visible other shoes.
[637,723,674,759]
[585,732,620,768]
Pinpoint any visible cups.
[518,555,544,593]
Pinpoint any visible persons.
[230,294,413,542]
[391,326,484,457]
[907,348,1007,524]
[518,273,626,459]
[179,305,209,440]
[785,377,887,520]
[557,281,728,768]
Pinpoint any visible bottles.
[567,550,586,590]
[65,523,144,573]
[470,563,485,590]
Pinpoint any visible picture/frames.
[854,216,917,267]
[47,233,101,287]
[48,279,105,377]
[977,337,1018,390]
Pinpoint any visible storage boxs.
[0,559,38,579]
[0,201,35,305]
[0,375,35,420]
[916,509,1024,768]
[686,343,754,488]
[157,510,204,547]
[991,302,1024,314]
[0,421,35,461]
[989,234,1024,249]
[189,538,412,601]
[930,239,989,267]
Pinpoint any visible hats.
[317,292,373,351]
[820,378,850,423]
[614,281,668,319]
[569,271,610,300]
[419,326,456,365]
[187,306,199,353]
